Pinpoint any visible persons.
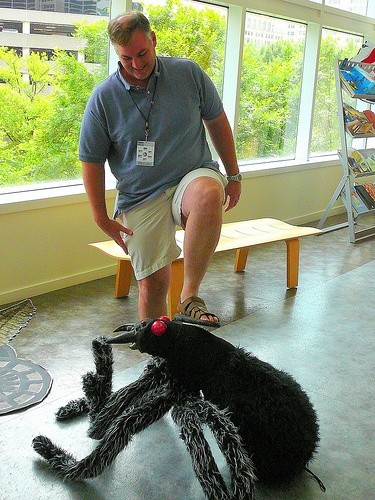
[78,11,242,328]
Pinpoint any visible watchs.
[226,173,242,182]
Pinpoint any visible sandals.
[173,296,220,328]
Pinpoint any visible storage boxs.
[343,185,375,213]
[349,65,375,95]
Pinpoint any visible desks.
[87,217,325,321]
